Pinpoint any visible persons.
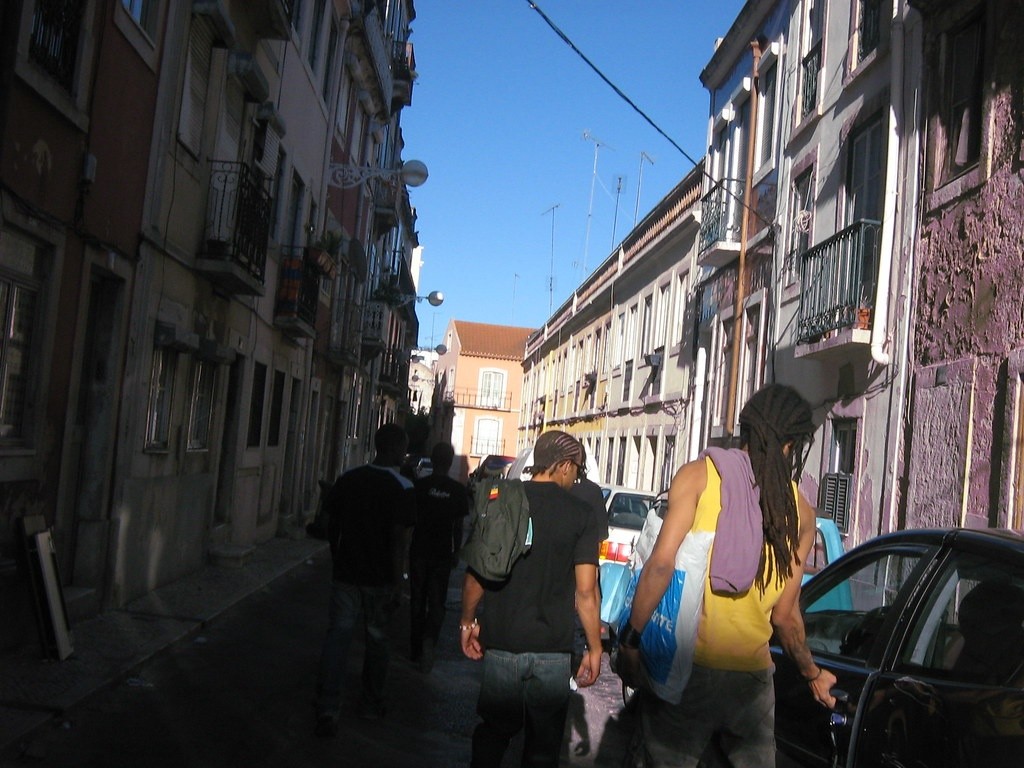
[570,442,610,678]
[318,421,413,736]
[407,441,467,663]
[622,382,837,768]
[460,430,601,768]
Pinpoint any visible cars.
[774,527,1024,768]
[600,484,854,610]
[416,458,433,478]
[478,455,515,479]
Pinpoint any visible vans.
[507,447,600,484]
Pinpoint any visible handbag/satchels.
[609,489,717,709]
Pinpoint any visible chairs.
[614,512,641,525]
[943,578,1024,685]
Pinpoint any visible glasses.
[571,460,584,476]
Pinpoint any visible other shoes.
[366,689,392,716]
[315,719,337,738]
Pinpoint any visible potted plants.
[207,233,234,262]
[307,230,353,280]
[846,296,874,330]
[283,250,304,281]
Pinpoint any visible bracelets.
[459,617,477,630]
[807,668,822,681]
[620,619,641,649]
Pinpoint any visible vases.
[645,355,661,366]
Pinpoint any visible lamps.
[396,291,445,307]
[328,159,428,190]
[408,343,447,355]
